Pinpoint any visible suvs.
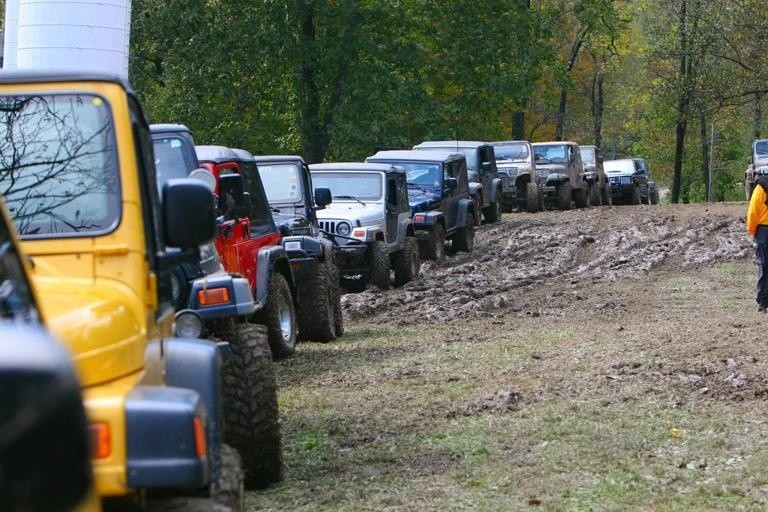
[744,140,768,200]
[487,141,657,211]
[307,140,504,294]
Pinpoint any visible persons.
[747,174,768,312]
